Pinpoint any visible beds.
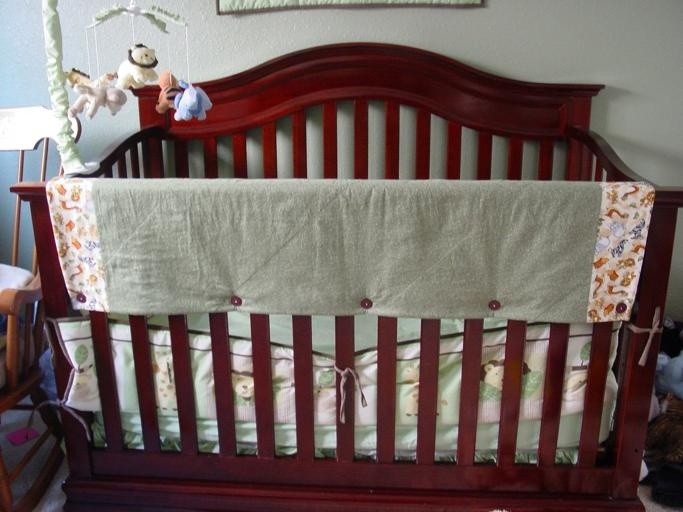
[11,42,680,512]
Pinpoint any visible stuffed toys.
[155,70,209,124]
[115,43,160,88]
[68,76,126,120]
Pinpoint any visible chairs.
[0,106,80,511]
